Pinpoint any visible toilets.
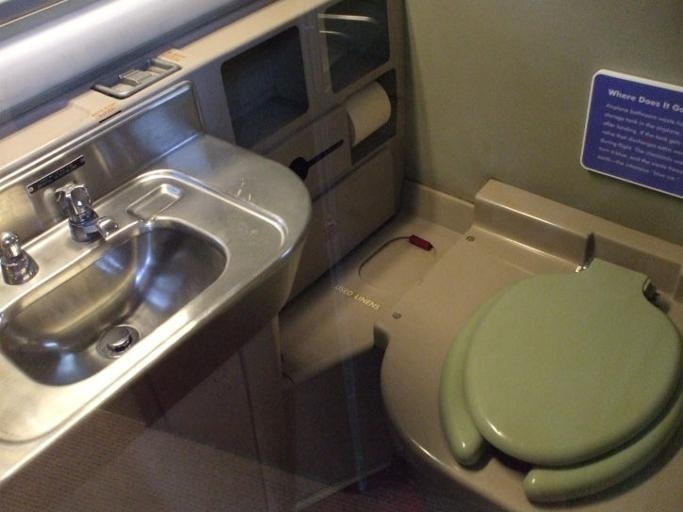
[379,180,680,510]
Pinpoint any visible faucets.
[54,180,120,242]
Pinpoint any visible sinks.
[0,214,230,389]
